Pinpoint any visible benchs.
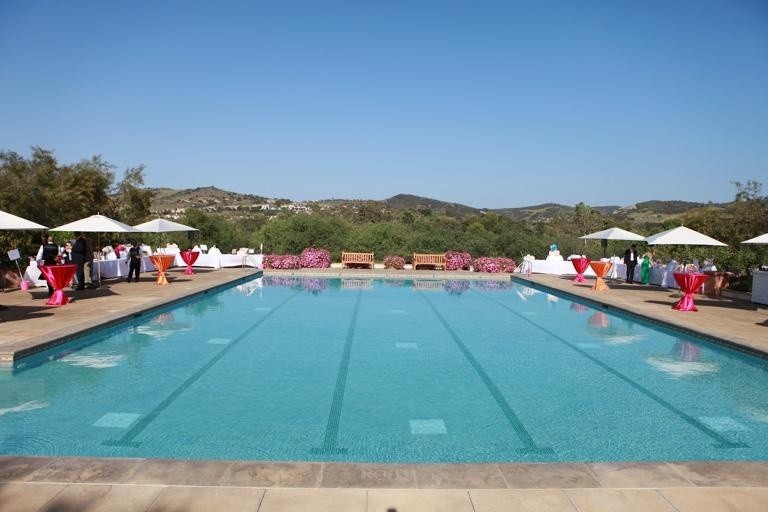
[413,252,448,272]
[340,278,373,290]
[413,280,447,292]
[342,251,374,271]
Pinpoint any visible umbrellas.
[645,224,727,250]
[47,212,140,286]
[0,210,50,233]
[740,230,768,246]
[130,218,200,251]
[578,225,646,256]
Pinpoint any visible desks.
[22,243,264,305]
[671,339,699,366]
[155,310,175,328]
[584,309,610,329]
[515,254,734,313]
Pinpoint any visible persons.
[124,240,143,283]
[35,236,62,297]
[623,244,637,284]
[81,235,94,285]
[71,231,90,290]
[62,242,73,263]
